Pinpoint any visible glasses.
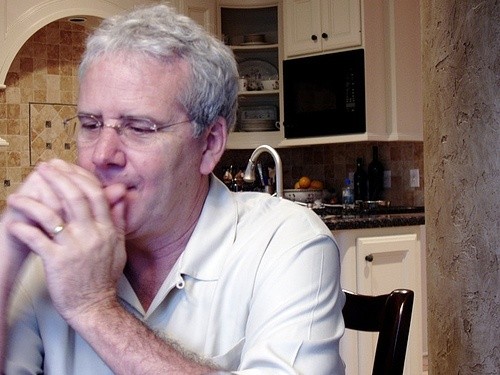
[63,114,196,151]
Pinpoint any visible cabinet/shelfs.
[329,226,428,375]
[179,0,424,149]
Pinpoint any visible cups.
[221,33,229,45]
[238,61,279,90]
[261,80,279,90]
[244,33,265,42]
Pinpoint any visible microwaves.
[281,48,365,139]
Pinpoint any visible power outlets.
[384,170,392,188]
[409,169,420,187]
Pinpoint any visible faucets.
[243,144,284,199]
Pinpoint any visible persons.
[0,3,345,375]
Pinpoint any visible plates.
[240,41,271,45]
[238,106,277,131]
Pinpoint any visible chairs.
[341,289,414,375]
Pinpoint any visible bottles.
[238,76,248,91]
[224,164,275,196]
[353,157,368,209]
[367,145,385,210]
[320,177,340,215]
[342,179,355,216]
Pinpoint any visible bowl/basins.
[284,188,322,203]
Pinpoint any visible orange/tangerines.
[294,176,322,190]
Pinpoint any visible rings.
[49,221,67,237]
